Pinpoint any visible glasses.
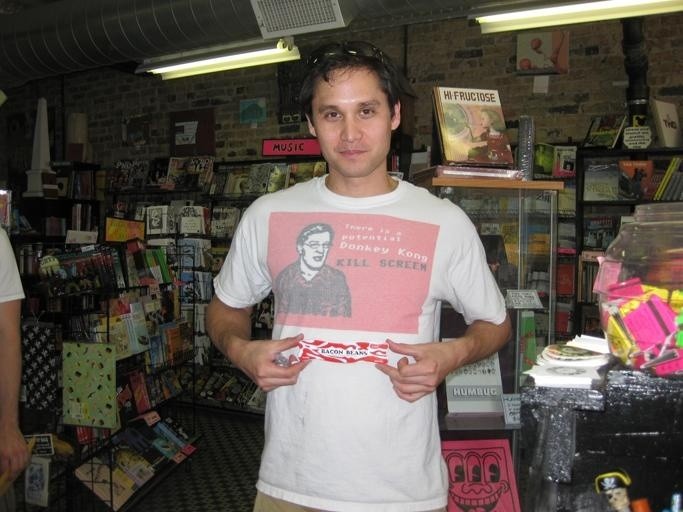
[308,41,387,64]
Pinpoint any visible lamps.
[134,37,300,81]
[467,1,682,34]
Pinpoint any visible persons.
[196,42,516,512]
[1,224,32,512]
[276,223,352,317]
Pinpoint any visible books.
[390,88,681,510]
[9,157,326,511]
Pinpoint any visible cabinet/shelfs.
[18,245,195,511]
[10,197,100,254]
[576,145,682,338]
[107,157,329,418]
